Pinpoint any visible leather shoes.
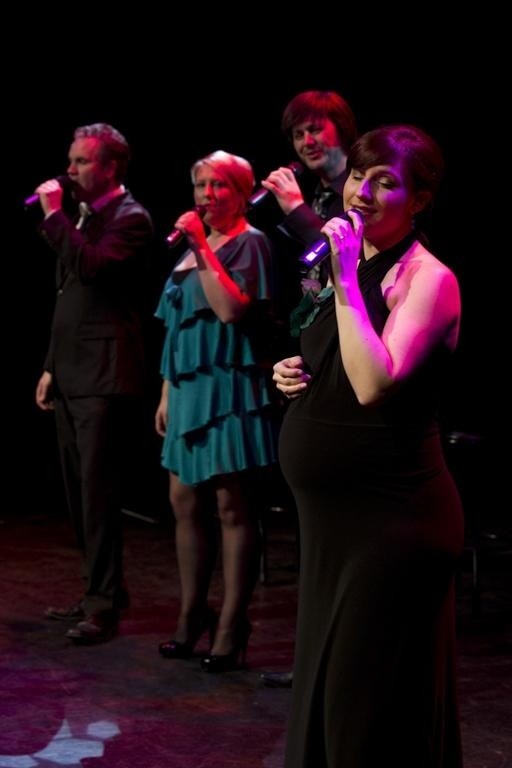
[63,621,109,640]
[44,600,86,619]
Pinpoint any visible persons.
[33,120,154,641]
[270,122,465,768]
[261,88,361,688]
[153,149,280,672]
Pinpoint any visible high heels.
[201,617,251,671]
[158,609,214,659]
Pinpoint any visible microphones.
[297,207,365,274]
[247,161,304,213]
[165,205,207,249]
[23,174,69,211]
